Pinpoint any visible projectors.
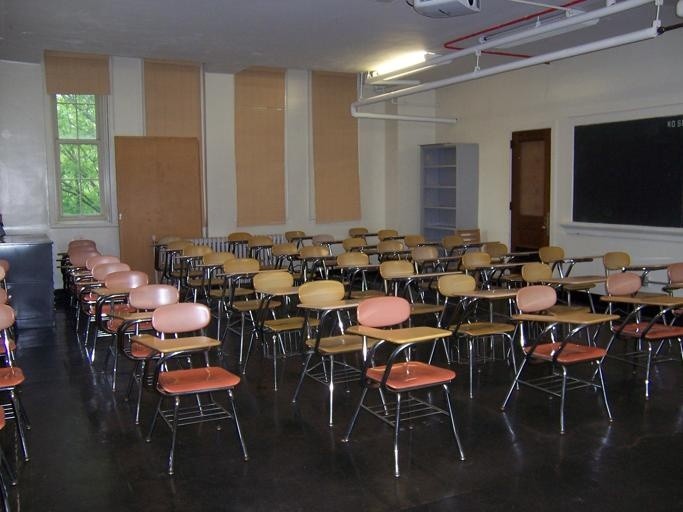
[413,0,481,18]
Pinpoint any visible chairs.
[57,228,681,478]
[0,256,30,512]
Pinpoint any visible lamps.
[363,51,442,85]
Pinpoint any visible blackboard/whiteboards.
[573,114,683,228]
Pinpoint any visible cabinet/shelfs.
[414,142,479,261]
[0,234,55,329]
